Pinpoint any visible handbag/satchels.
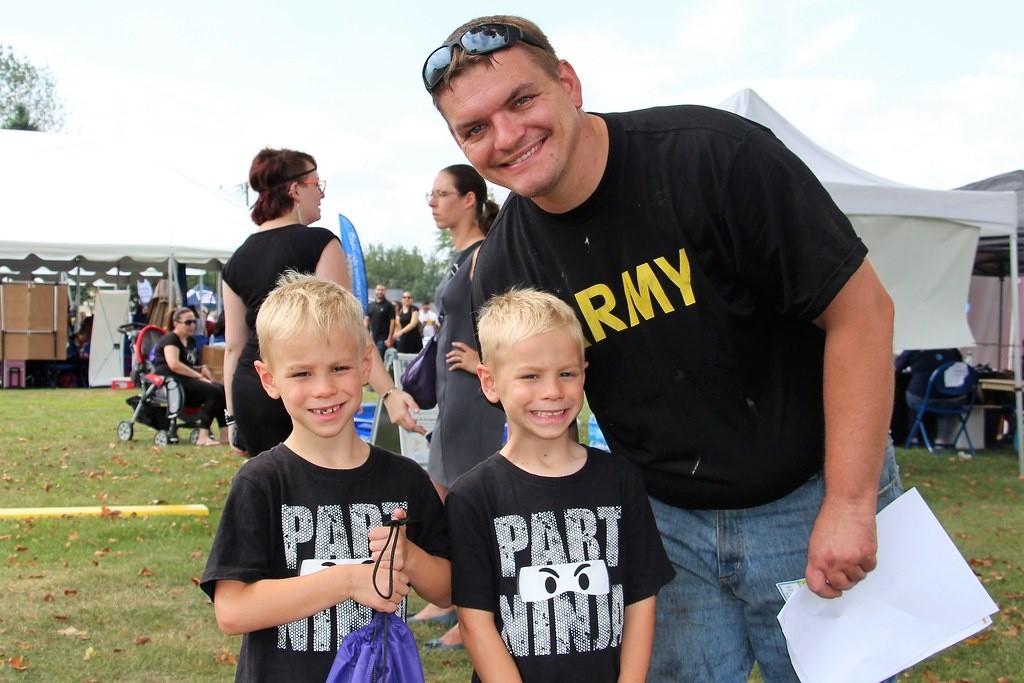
[325,610,426,683]
[400,338,439,410]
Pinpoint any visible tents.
[1,129,258,332]
[0,241,253,354]
[715,84,1024,473]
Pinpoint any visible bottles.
[964,352,973,366]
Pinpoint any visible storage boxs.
[202,345,226,381]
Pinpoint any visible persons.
[151,17,896,683]
[893,349,972,452]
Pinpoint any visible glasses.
[290,179,327,198]
[422,24,550,93]
[176,320,197,326]
[426,190,460,199]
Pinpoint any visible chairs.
[904,363,979,454]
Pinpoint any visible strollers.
[116,321,231,447]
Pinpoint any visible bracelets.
[381,387,397,401]
[223,408,236,427]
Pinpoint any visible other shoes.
[425,639,464,649]
[406,607,457,626]
[195,438,220,446]
